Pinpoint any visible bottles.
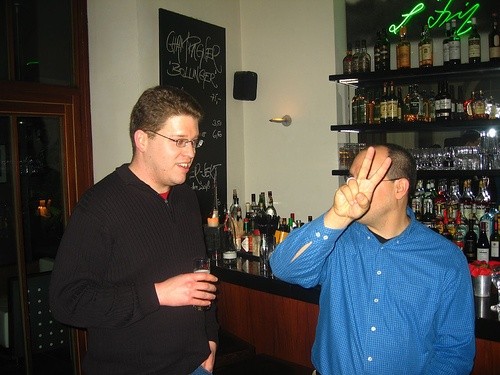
[340,83,500,323]
[351,40,361,73]
[417,22,434,67]
[488,13,500,62]
[374,32,381,71]
[342,42,353,75]
[358,40,371,74]
[449,18,461,65]
[380,27,390,71]
[395,27,411,70]
[468,18,481,65]
[217,188,314,263]
[442,21,451,66]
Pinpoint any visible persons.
[269,143,476,375]
[47,85,218,375]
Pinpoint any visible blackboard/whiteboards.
[158,8,227,226]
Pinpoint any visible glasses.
[344,173,409,187]
[143,130,203,148]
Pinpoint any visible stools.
[214,330,315,375]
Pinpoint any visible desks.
[215,254,500,375]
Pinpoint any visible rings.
[347,177,355,184]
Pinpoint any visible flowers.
[468,260,499,277]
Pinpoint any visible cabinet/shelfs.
[326,61,500,175]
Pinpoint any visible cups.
[193,258,210,312]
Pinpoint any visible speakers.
[233,71,258,101]
